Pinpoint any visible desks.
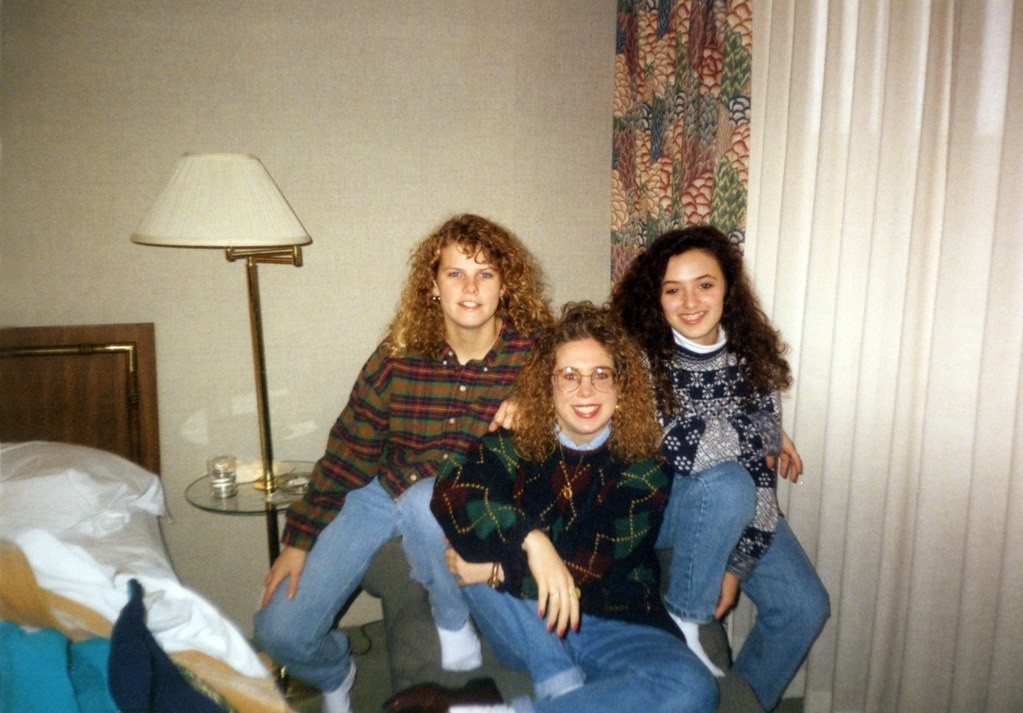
[185,449,326,618]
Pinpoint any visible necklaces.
[553,438,599,501]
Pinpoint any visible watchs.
[487,554,501,591]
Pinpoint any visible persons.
[430,299,720,713]
[608,223,834,713]
[251,211,556,713]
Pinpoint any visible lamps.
[133,149,316,491]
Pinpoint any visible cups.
[207,451,237,498]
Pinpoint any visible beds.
[0,317,297,712]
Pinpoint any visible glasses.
[550,366,619,393]
